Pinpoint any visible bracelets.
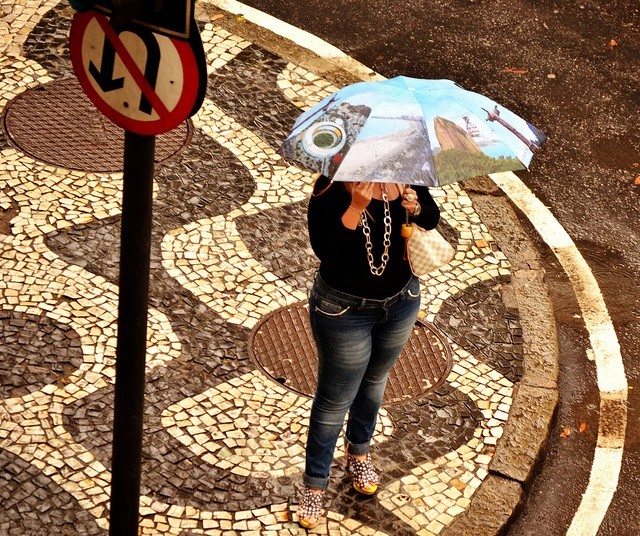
[349,205,362,213]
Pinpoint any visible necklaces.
[358,182,392,276]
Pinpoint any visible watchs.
[408,202,421,216]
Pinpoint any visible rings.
[406,193,415,201]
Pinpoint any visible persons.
[296,174,441,529]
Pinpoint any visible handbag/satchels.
[402,184,456,278]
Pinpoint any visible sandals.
[347,452,382,496]
[298,484,326,529]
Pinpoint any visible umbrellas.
[275,75,551,237]
